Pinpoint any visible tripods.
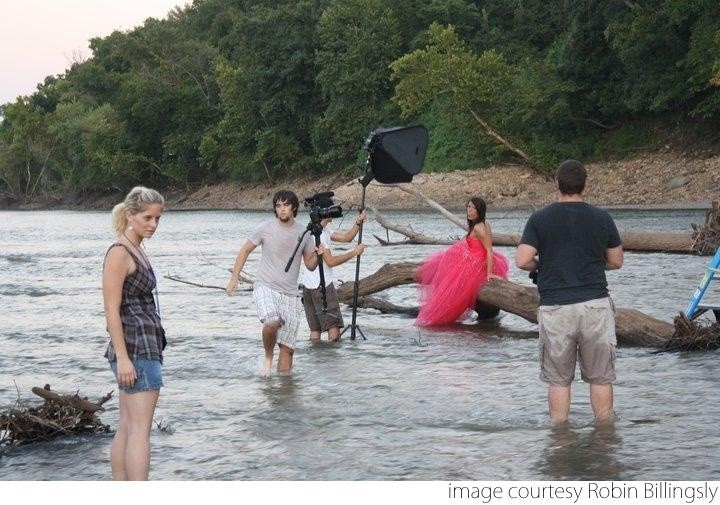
[330,188,368,342]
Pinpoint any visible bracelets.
[356,220,361,226]
[354,248,360,256]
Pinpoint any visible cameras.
[304,199,344,231]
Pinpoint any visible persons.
[412,195,508,329]
[225,189,325,381]
[513,158,623,424]
[300,209,369,342]
[101,186,169,480]
